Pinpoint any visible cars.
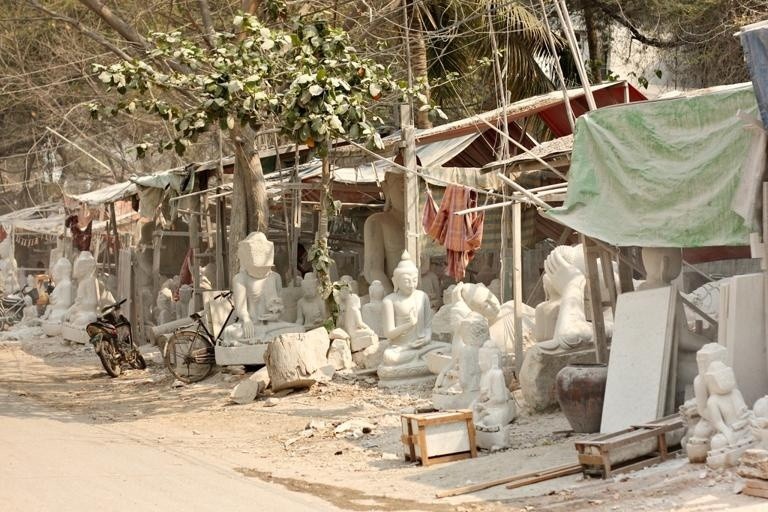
[4,286,54,316]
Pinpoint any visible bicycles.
[0,285,34,332]
[162,291,239,384]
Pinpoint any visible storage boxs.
[401,410,478,466]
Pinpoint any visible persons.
[175,282,192,320]
[380,248,450,365]
[42,257,73,321]
[362,147,442,304]
[700,358,756,449]
[470,338,518,428]
[222,229,307,345]
[295,270,329,332]
[455,309,505,393]
[154,287,173,327]
[451,279,535,358]
[677,339,729,440]
[359,278,386,341]
[342,292,379,353]
[542,240,646,350]
[636,245,716,398]
[66,249,101,327]
[17,294,40,329]
[0,234,20,295]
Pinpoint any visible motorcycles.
[86,299,146,378]
